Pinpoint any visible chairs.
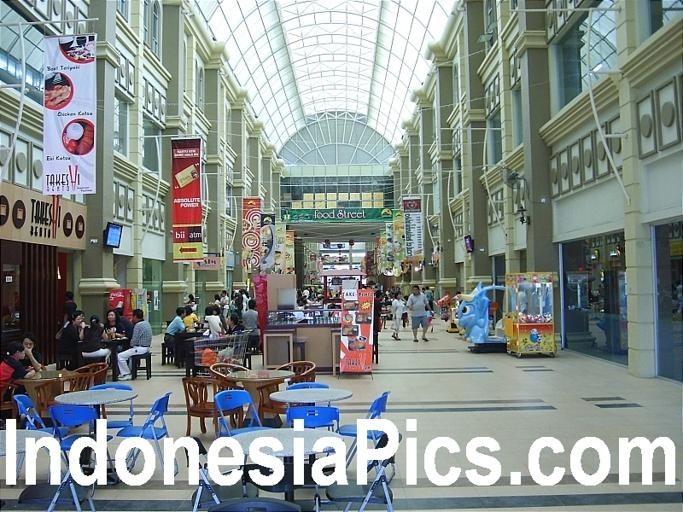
[0,329,401,512]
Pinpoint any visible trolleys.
[192,329,256,379]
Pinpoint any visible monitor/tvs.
[464,235,473,252]
[103,222,123,248]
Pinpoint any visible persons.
[297,284,435,342]
[0,291,261,431]
[456,291,463,306]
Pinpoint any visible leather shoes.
[118,374,133,381]
